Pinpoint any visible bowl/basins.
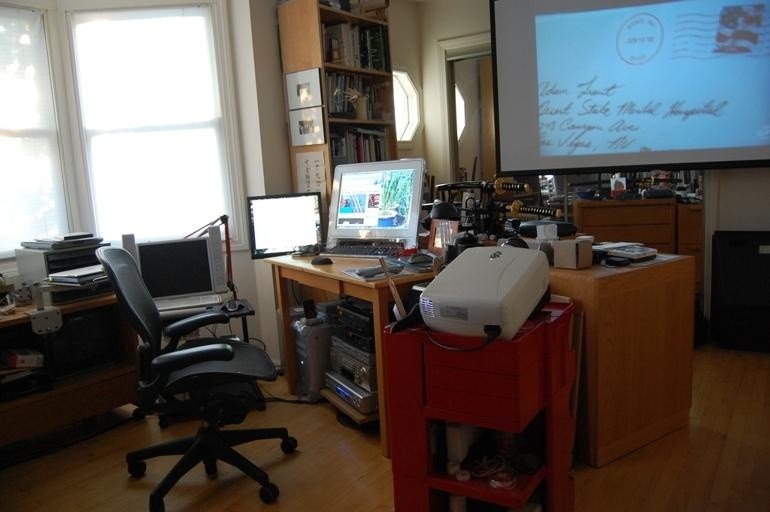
[577,192,593,199]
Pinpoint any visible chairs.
[88,242,300,512]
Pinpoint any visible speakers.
[207,225,228,294]
[710,230,770,354]
[121,234,137,265]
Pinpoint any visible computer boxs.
[288,310,345,402]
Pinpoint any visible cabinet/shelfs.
[542,238,700,469]
[377,292,583,512]
[569,196,707,342]
[274,0,401,243]
[0,285,145,467]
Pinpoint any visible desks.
[258,235,513,461]
[168,294,257,343]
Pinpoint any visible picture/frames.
[427,217,460,259]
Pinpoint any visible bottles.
[16,278,44,311]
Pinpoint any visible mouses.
[311,257,333,265]
[226,301,238,311]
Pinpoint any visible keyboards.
[153,294,224,311]
[319,244,403,258]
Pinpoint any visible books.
[0,367,33,384]
[319,1,396,165]
[19,233,104,284]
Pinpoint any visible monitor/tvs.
[246,191,322,260]
[325,159,426,250]
[137,237,215,300]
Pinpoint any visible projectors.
[419,245,549,340]
